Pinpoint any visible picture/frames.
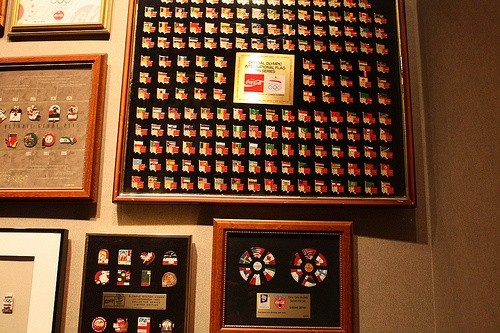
[0,226,70,332]
[0,52,109,206]
[7,0,115,41]
[0,0,9,37]
[77,230,194,333]
[208,215,355,332]
[110,0,417,208]
